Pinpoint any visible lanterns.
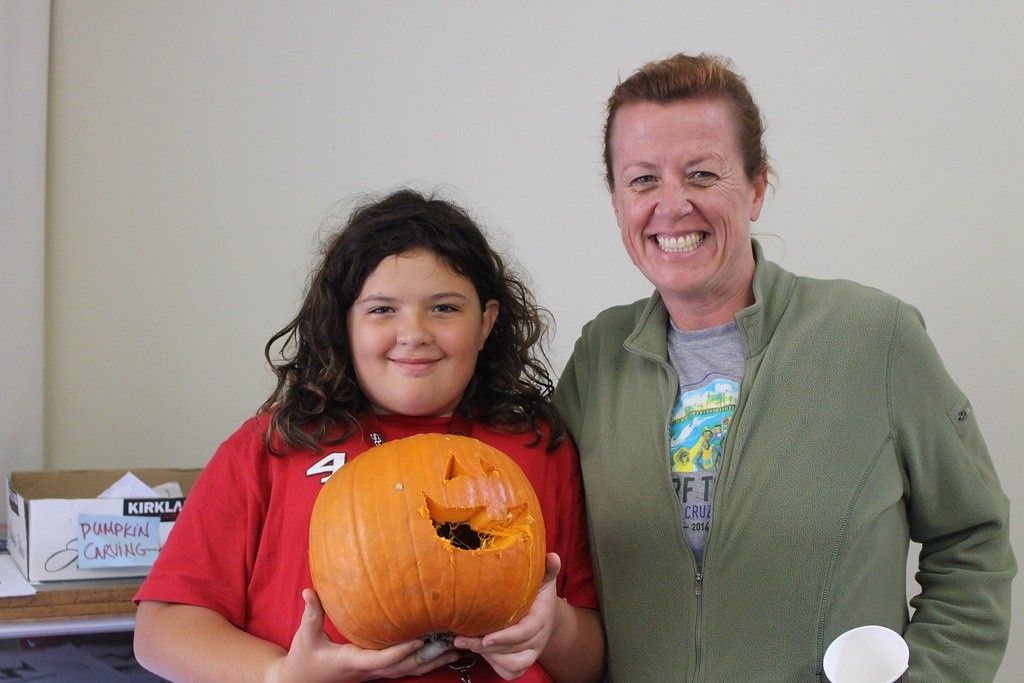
[309,432,547,663]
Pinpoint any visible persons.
[132,188,606,681]
[548,52,1019,683]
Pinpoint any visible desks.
[0,551,138,639]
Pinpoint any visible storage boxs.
[6,470,203,583]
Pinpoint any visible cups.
[822,626,911,683]
